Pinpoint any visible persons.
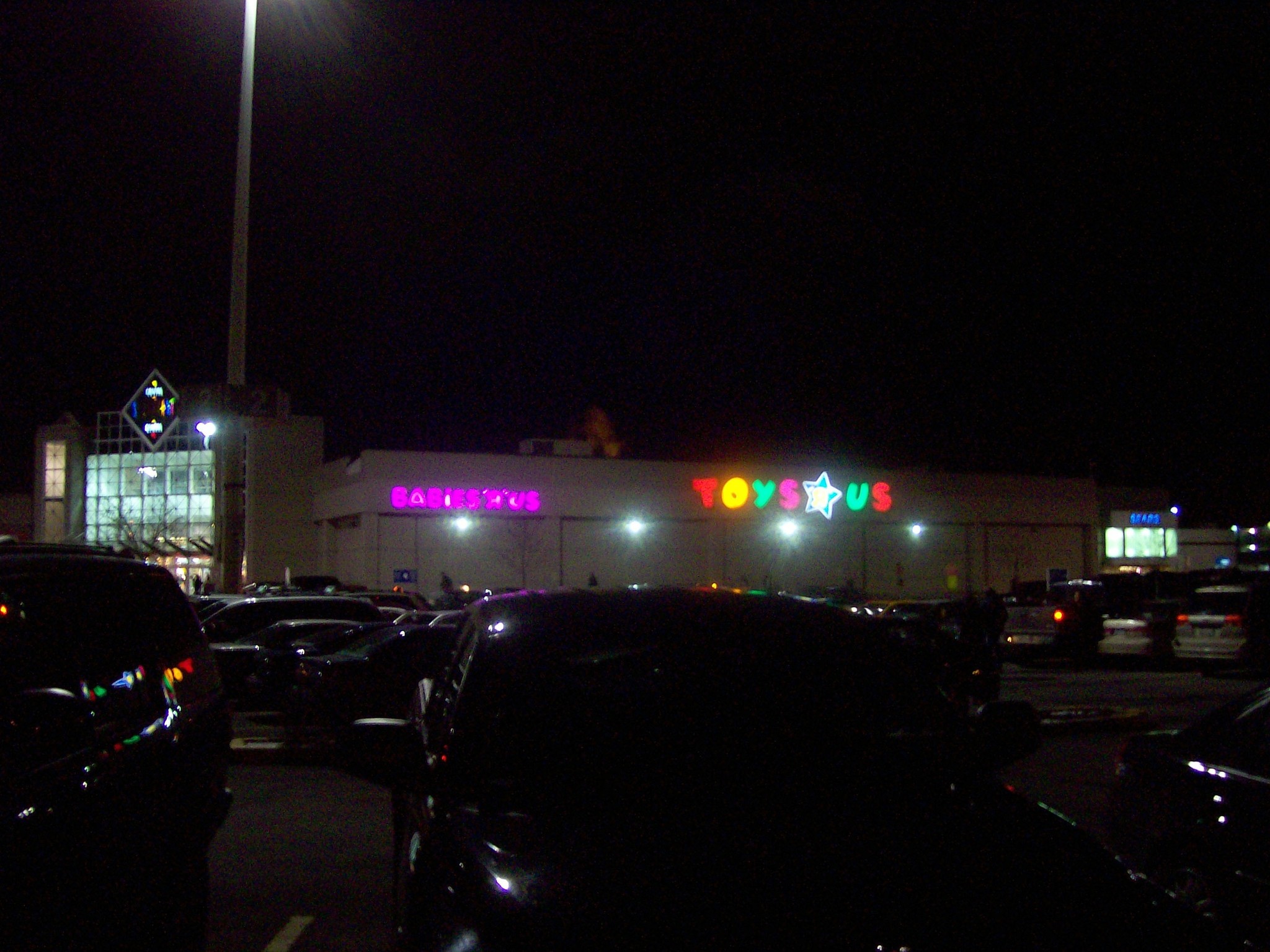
[436,574,474,611]
[193,575,203,594]
[955,584,1007,654]
[1063,589,1093,667]
[838,578,863,607]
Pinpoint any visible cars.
[0,541,1189,951]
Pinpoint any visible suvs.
[1169,584,1256,660]
